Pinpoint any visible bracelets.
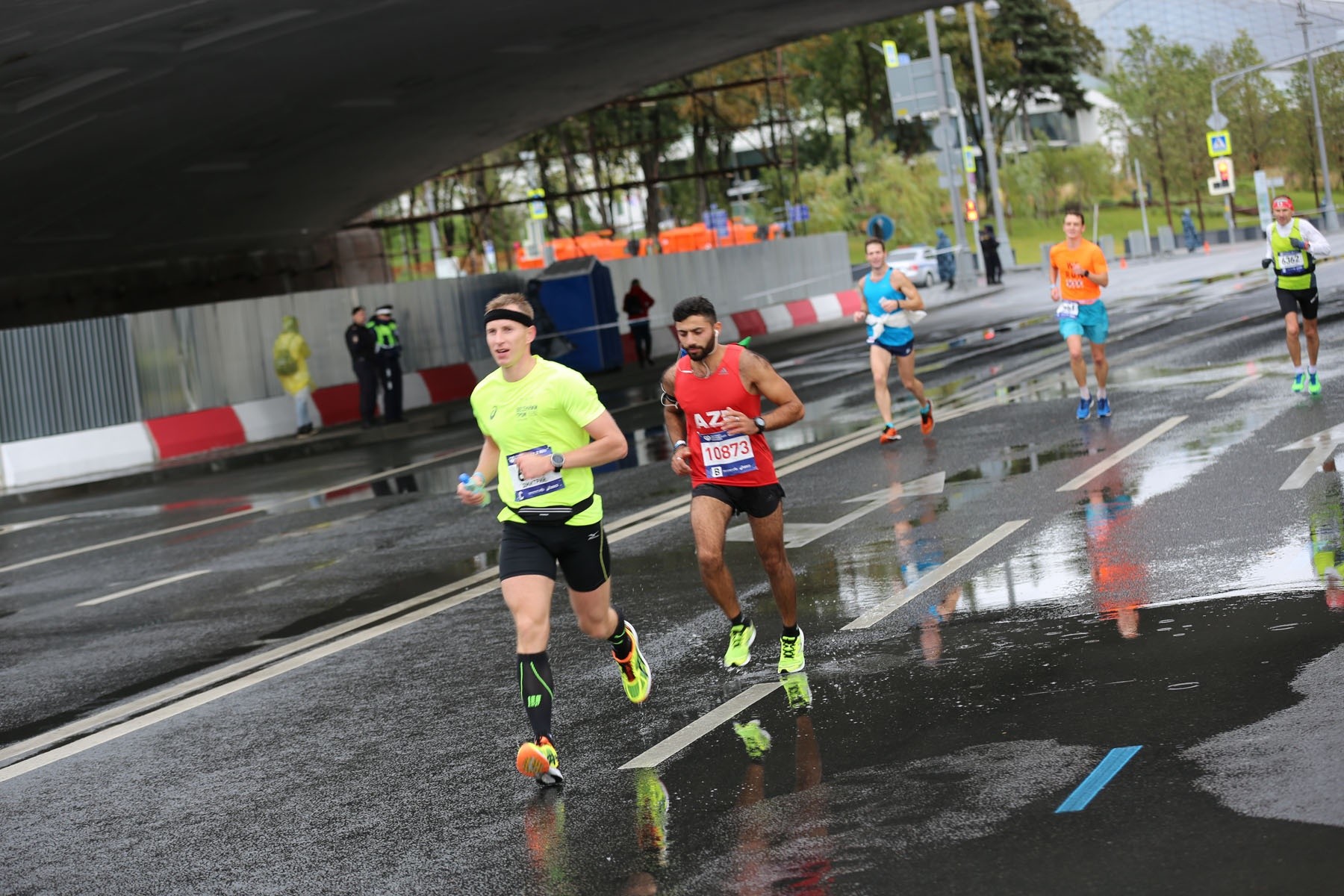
[673,440,687,449]
[1084,269,1090,276]
[470,470,485,488]
[1052,285,1056,288]
[896,299,901,309]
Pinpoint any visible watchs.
[550,453,565,472]
[752,416,766,435]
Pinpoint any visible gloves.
[1289,237,1308,250]
[1262,258,1272,268]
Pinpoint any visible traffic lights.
[1207,157,1235,196]
[965,200,979,222]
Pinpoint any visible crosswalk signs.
[527,188,548,221]
[1206,129,1232,157]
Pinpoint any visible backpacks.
[276,333,303,375]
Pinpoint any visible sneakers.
[734,725,772,757]
[880,425,901,444]
[515,731,564,786]
[635,777,671,868]
[1075,392,1095,421]
[779,671,813,713]
[920,399,936,434]
[722,616,757,667]
[1292,372,1306,391]
[1096,396,1111,417]
[777,623,805,673]
[1307,366,1322,395]
[611,619,652,703]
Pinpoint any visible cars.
[886,242,941,288]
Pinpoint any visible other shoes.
[386,415,408,424]
[296,421,319,439]
[362,418,384,428]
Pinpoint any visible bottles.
[459,472,491,508]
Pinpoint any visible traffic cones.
[1119,257,1127,269]
[1203,241,1210,253]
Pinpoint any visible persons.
[457,292,653,786]
[1048,211,1112,422]
[934,225,1002,288]
[622,279,655,369]
[272,315,324,439]
[1263,196,1333,395]
[883,439,1343,662]
[344,306,411,429]
[854,237,935,444]
[530,670,812,896]
[659,297,805,675]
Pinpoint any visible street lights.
[939,0,1016,270]
[1292,1,1341,234]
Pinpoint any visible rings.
[676,457,682,459]
[736,416,739,421]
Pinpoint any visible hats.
[376,305,393,316]
[352,307,364,314]
